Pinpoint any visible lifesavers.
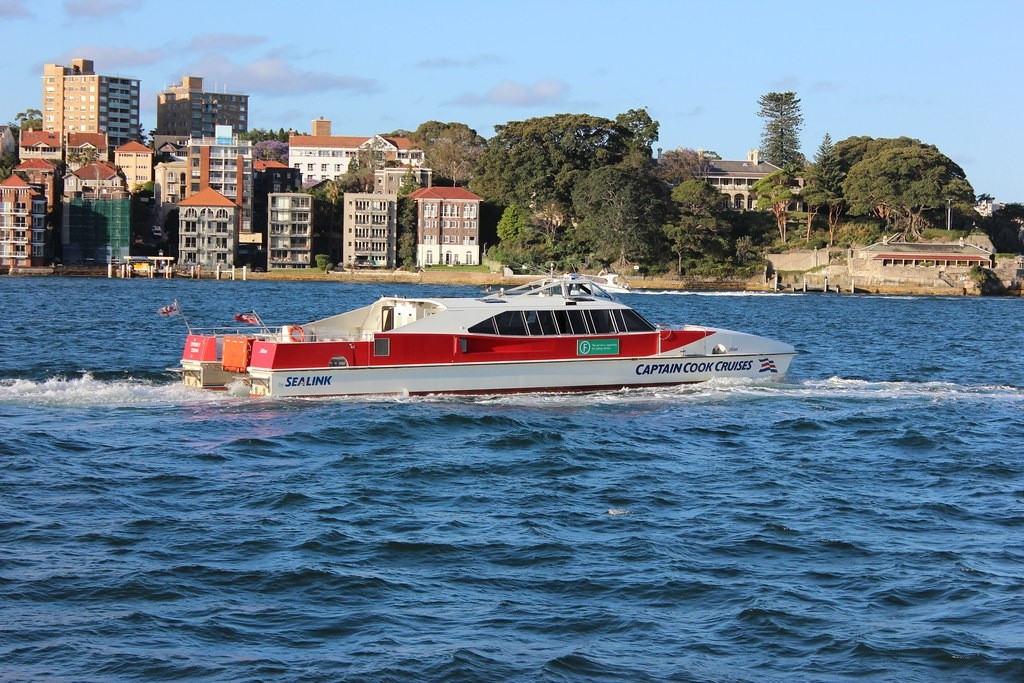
[288,324,305,342]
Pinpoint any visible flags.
[234,312,261,326]
[156,304,179,317]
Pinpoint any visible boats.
[177,259,800,409]
